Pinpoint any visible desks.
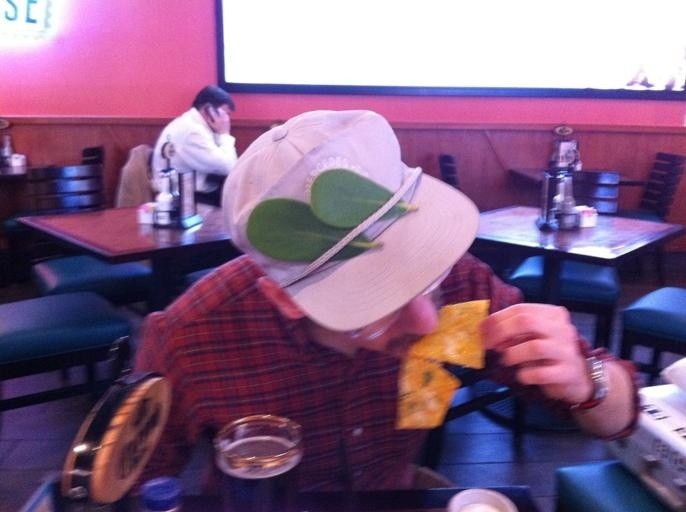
[478,203,686,435]
[11,200,232,310]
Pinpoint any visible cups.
[211,412,305,512]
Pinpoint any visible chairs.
[513,169,623,353]
[612,152,685,290]
[19,146,153,389]
[1,293,144,413]
[437,152,457,192]
[621,287,685,382]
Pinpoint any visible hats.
[218,108,483,336]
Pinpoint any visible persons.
[122,105,645,501]
[147,82,238,206]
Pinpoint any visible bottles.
[152,192,175,227]
[1,134,12,166]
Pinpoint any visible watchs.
[563,346,613,412]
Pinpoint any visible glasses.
[340,262,455,345]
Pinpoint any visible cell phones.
[205,102,219,123]
